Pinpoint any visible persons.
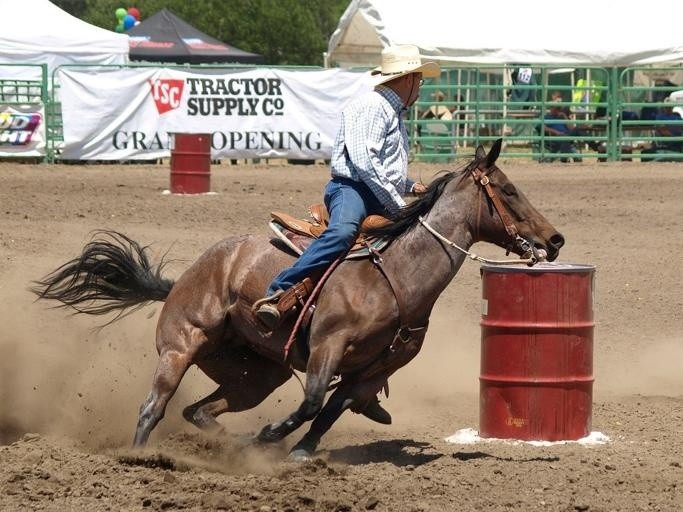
[420,89,453,122]
[450,93,469,128]
[251,44,443,333]
[653,96,682,155]
[535,91,601,161]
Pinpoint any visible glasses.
[403,74,425,87]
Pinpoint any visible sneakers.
[255,300,282,331]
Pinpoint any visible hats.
[359,43,442,87]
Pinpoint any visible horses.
[28,137,566,464]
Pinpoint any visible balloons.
[113,8,142,33]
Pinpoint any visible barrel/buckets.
[167,129,213,195]
[478,262,596,441]
